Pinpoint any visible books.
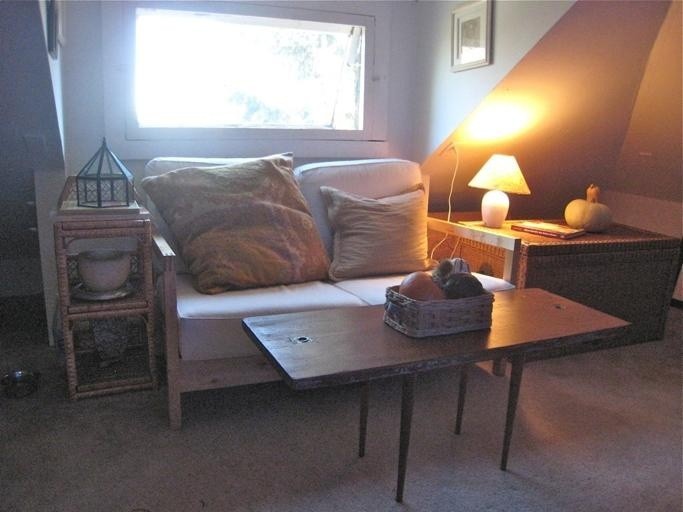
[510,220,588,240]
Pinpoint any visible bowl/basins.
[0,367,40,399]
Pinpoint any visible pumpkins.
[563,199,612,233]
[400,272,443,302]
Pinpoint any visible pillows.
[319,186,428,281]
[140,153,331,293]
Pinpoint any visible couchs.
[146,158,522,432]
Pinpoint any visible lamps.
[467,154,532,231]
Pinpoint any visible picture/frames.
[446,2,493,74]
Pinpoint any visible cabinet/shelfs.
[48,172,161,402]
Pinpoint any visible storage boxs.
[458,218,683,365]
